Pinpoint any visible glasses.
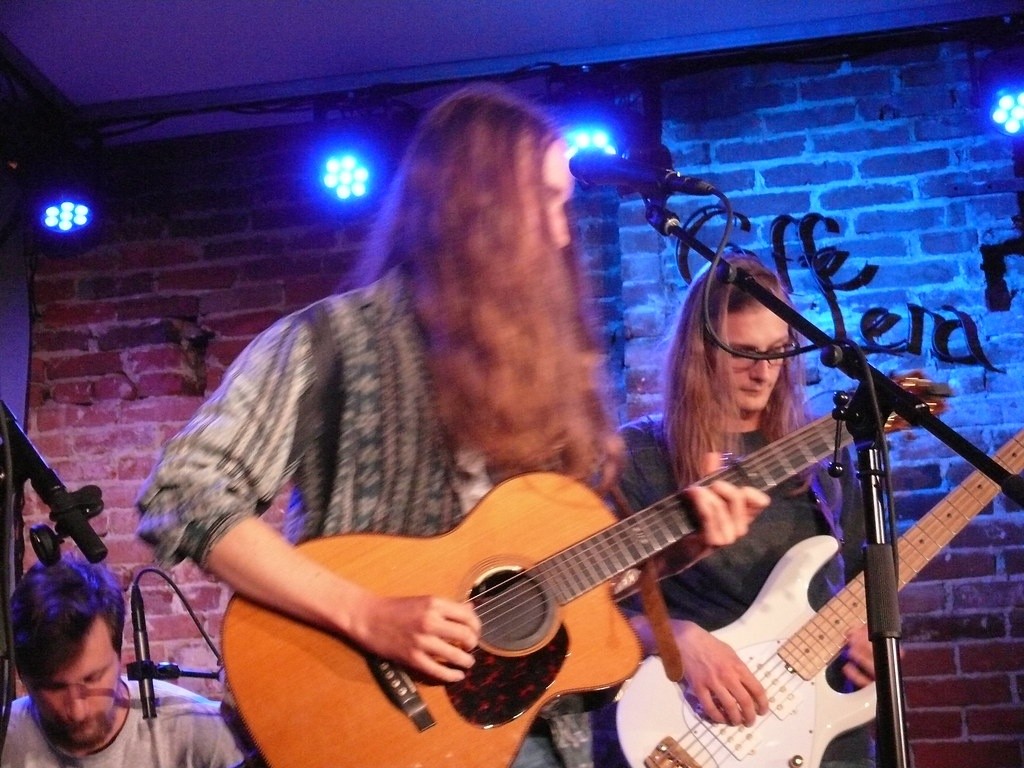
[724,331,802,370]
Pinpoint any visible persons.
[590,255,904,768]
[138,88,771,768]
[0,555,249,768]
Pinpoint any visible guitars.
[215,368,960,768]
[612,421,1024,768]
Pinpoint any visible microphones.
[568,147,716,197]
[131,584,158,717]
